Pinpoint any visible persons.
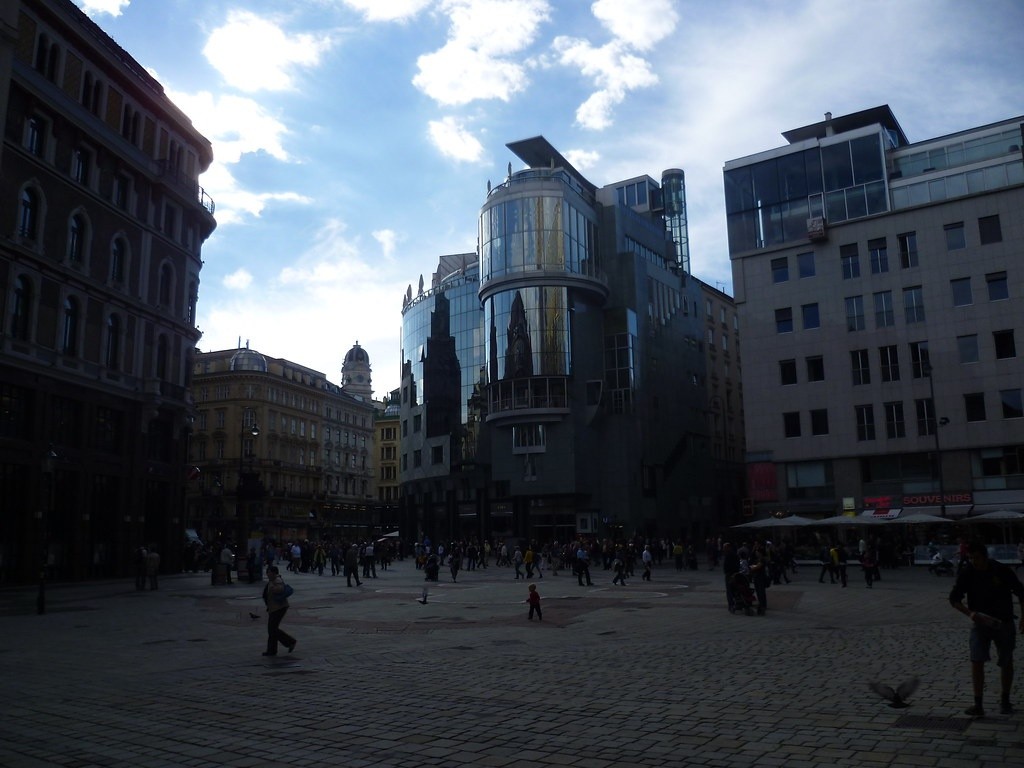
[526,584,543,621]
[182,537,408,586]
[705,536,799,617]
[261,565,297,656]
[894,530,1024,575]
[414,535,697,586]
[949,541,1024,715]
[817,536,881,589]
[137,544,160,591]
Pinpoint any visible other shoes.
[288,639,297,653]
[831,581,838,584]
[1000,703,1012,714]
[262,652,277,656]
[357,582,363,586]
[347,585,352,587]
[818,580,826,583]
[965,707,985,716]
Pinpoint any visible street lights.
[233,408,260,568]
[37,443,60,613]
[211,477,224,586]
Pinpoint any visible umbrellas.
[959,510,1024,524]
[890,513,957,523]
[729,514,889,528]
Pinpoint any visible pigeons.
[417,595,428,605]
[868,676,920,709]
[248,612,260,619]
